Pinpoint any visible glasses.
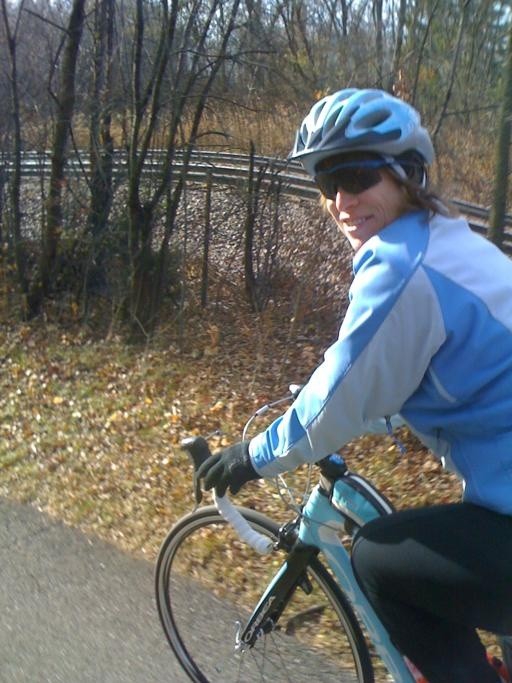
[313,158,421,198]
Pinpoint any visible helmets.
[286,88,434,179]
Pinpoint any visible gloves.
[195,440,263,497]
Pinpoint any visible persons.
[192,84,512,683]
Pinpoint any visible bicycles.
[155,384,512,683]
